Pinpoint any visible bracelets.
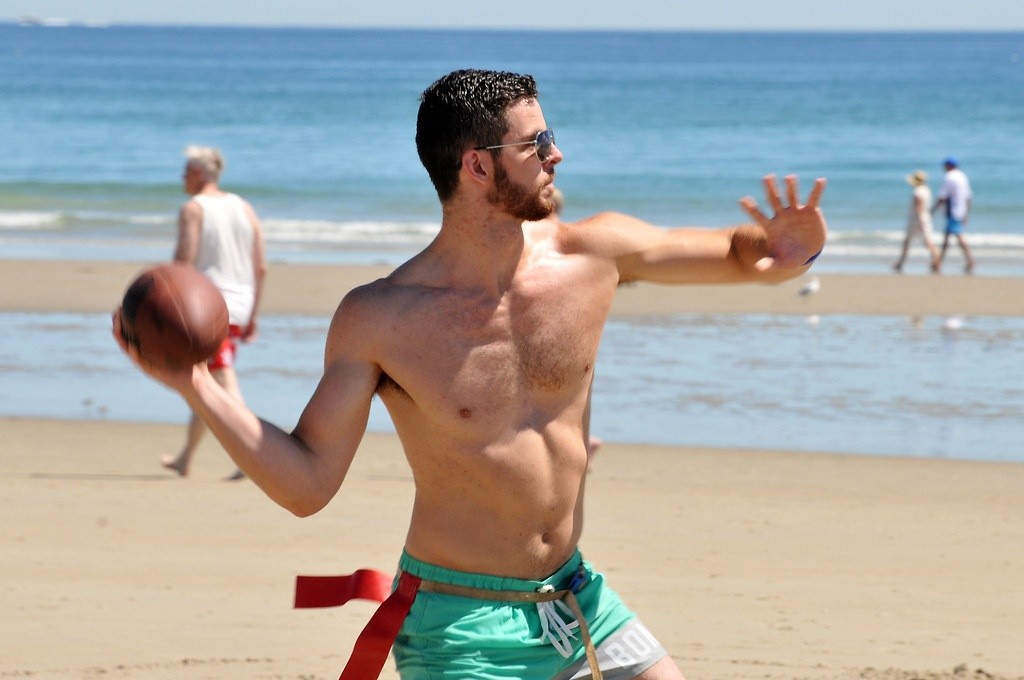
[802,249,823,266]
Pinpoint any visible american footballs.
[117,259,230,368]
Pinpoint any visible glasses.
[455,130,555,173]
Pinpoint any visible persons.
[891,172,939,270]
[111,69,828,680]
[929,157,977,271]
[161,144,267,480]
